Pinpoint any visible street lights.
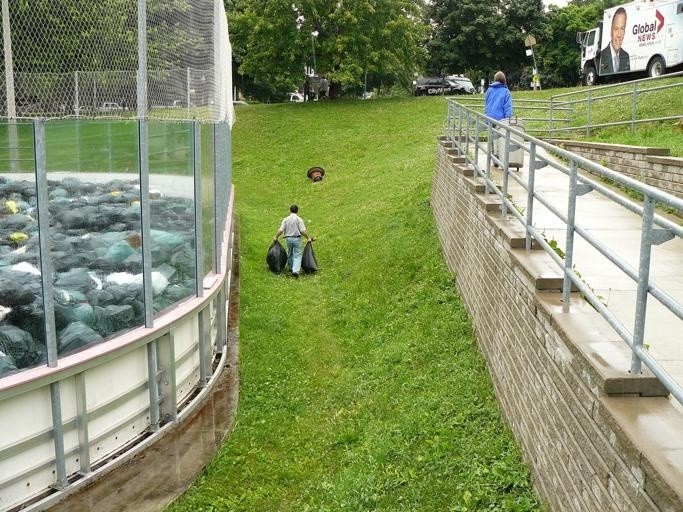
[311,30,319,102]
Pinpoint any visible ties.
[614,56,619,72]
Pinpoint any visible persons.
[302,76,332,104]
[599,7,632,76]
[483,70,513,168]
[271,203,312,279]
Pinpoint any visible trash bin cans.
[495,116,525,172]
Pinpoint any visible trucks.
[575,0,683,86]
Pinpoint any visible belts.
[283,236,300,238]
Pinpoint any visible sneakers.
[292,272,299,277]
[494,155,499,167]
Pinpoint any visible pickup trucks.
[153,99,195,108]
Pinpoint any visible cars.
[74,101,129,112]
[233,100,248,105]
[414,73,475,96]
[286,92,304,102]
